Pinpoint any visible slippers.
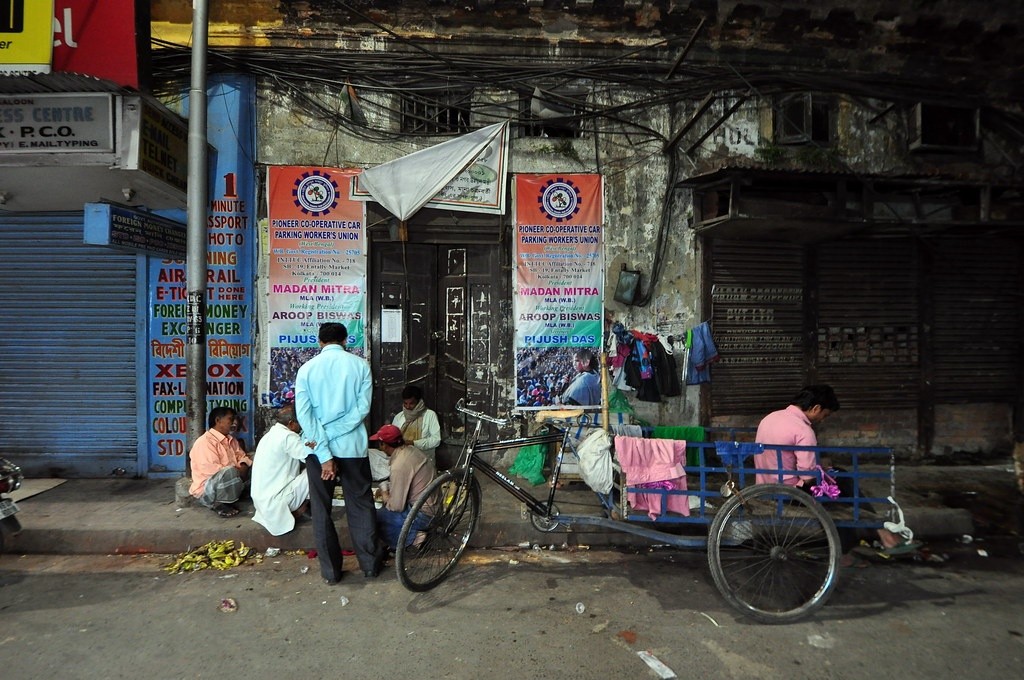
[210,504,237,518]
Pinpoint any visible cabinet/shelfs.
[548,413,632,489]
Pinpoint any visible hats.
[368,424,401,444]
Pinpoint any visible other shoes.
[891,548,932,565]
[292,511,312,522]
[364,562,383,577]
[407,533,431,559]
[324,576,339,585]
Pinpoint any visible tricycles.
[393,396,899,626]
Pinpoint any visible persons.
[516,346,602,407]
[188,406,252,516]
[250,387,443,552]
[753,385,923,554]
[295,322,385,585]
